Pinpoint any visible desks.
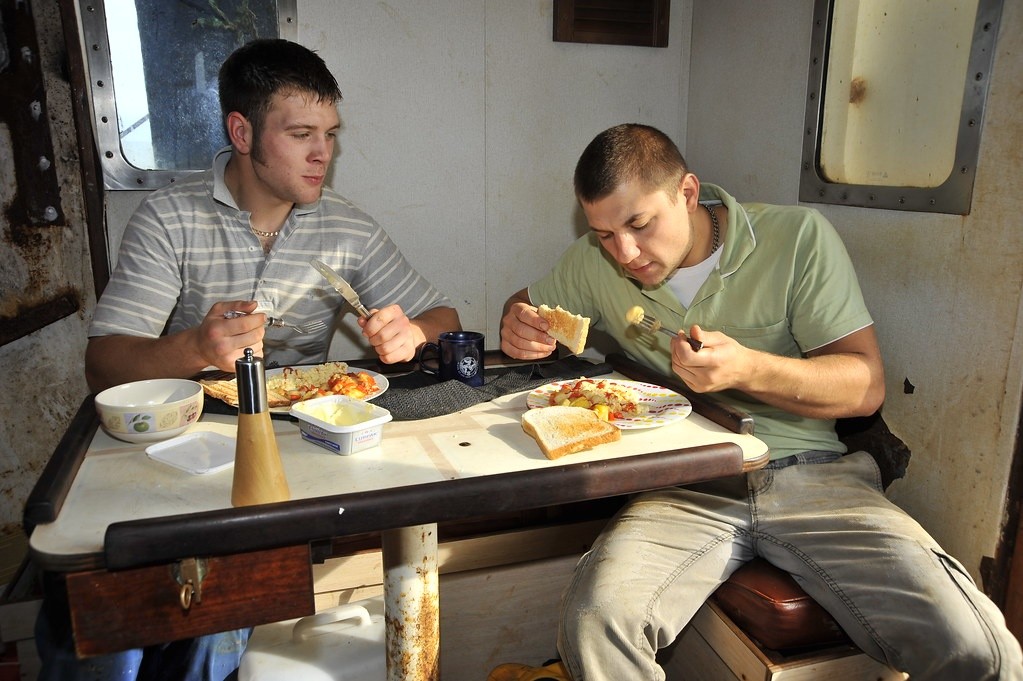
[21,349,767,681]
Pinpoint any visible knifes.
[310,258,372,321]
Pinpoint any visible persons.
[37,38,463,681]
[498,123,1023,681]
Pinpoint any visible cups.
[418,331,485,388]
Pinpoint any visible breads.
[522,407,621,460]
[537,305,591,356]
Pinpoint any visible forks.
[224,310,328,335]
[636,314,703,352]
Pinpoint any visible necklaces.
[251,227,280,237]
[702,203,720,256]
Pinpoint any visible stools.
[660,551,910,680]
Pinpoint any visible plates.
[224,365,389,415]
[527,379,692,429]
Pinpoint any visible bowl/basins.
[95,378,204,443]
